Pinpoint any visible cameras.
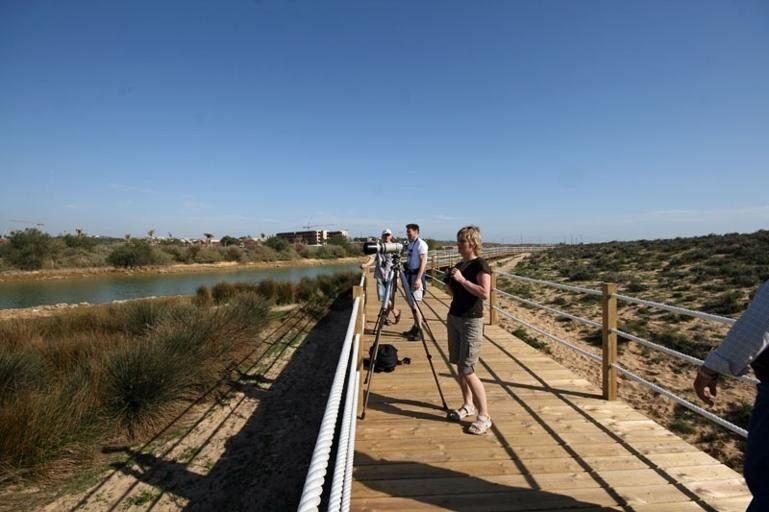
[363,240,409,255]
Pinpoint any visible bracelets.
[696,366,719,379]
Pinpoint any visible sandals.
[447,404,475,421]
[467,415,492,434]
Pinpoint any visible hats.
[382,229,392,235]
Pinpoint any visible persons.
[443,226,494,434]
[401,224,429,341]
[693,279,769,512]
[360,229,401,325]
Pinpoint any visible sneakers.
[403,325,417,336]
[385,320,392,326]
[395,310,400,323]
[408,327,422,341]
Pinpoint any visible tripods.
[362,255,448,419]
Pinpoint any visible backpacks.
[369,343,410,373]
